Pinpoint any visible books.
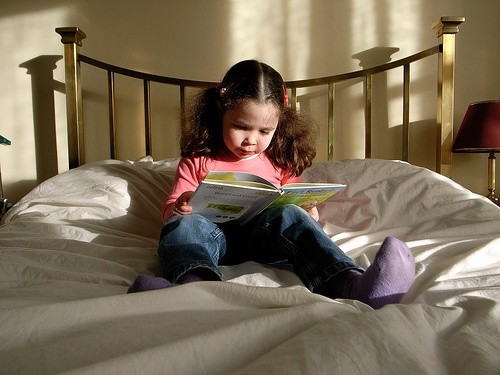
[187,170,348,228]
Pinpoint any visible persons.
[125,59,416,309]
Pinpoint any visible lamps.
[452,99,500,208]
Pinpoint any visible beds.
[0,14,500,375]
